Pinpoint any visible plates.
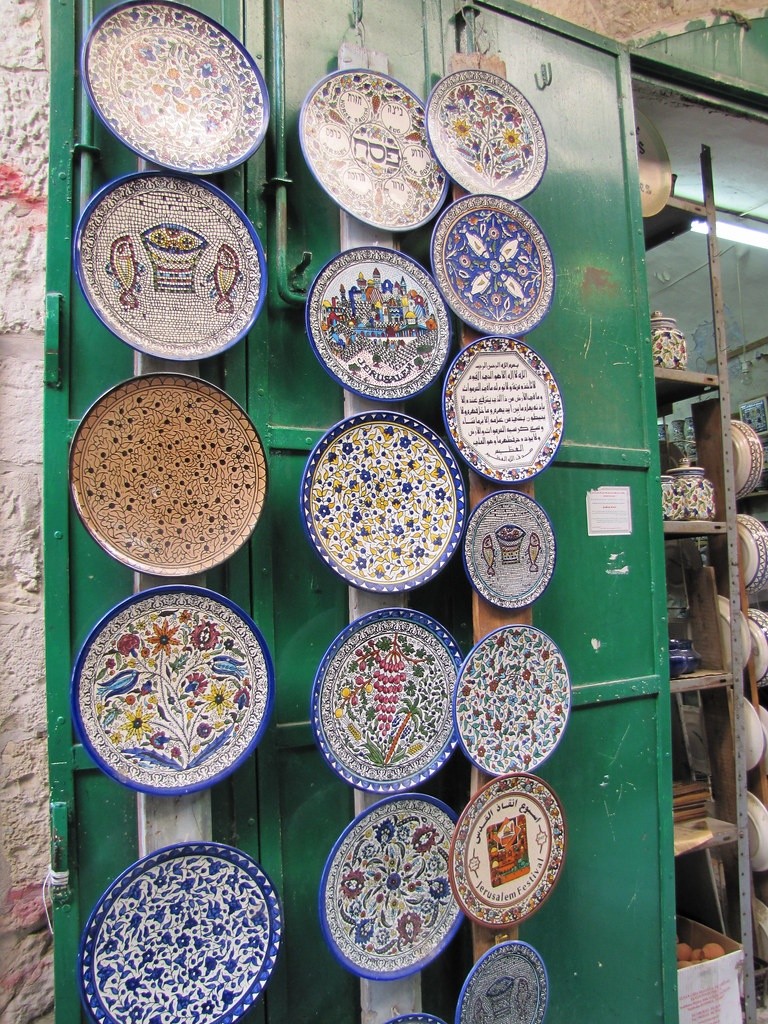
[684,417,767,958]
[72,0,573,1024]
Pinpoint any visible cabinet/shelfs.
[639,145,758,1024]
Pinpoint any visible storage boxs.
[675,915,744,1024]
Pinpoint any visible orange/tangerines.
[676,941,725,969]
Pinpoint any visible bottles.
[661,458,717,523]
[649,311,688,373]
